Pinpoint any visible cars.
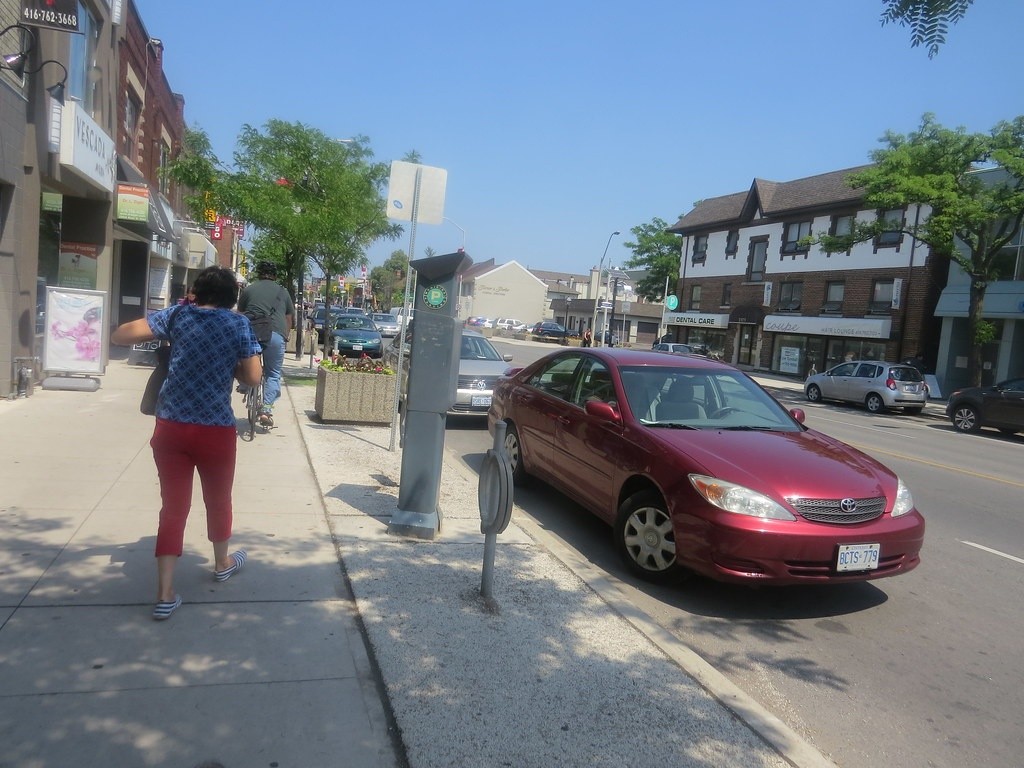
[383,318,513,418]
[649,343,696,355]
[329,313,384,359]
[803,358,930,418]
[486,344,926,588]
[946,377,1024,435]
[463,316,534,335]
[594,330,621,344]
[305,303,416,345]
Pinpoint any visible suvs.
[532,320,579,338]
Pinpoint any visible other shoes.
[214,550,246,581]
[260,411,274,426]
[153,594,182,619]
[237,385,254,395]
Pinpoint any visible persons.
[537,326,543,334]
[707,352,721,362]
[583,328,591,347]
[235,258,296,427]
[110,265,261,616]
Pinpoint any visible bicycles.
[238,343,271,439]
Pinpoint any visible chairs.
[603,372,645,421]
[655,377,708,423]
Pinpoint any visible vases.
[313,364,396,424]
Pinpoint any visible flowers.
[313,350,395,375]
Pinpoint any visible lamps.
[0,25,36,79]
[0,61,68,107]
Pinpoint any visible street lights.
[561,296,572,344]
[589,230,621,348]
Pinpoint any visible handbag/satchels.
[244,312,273,341]
[140,305,186,415]
